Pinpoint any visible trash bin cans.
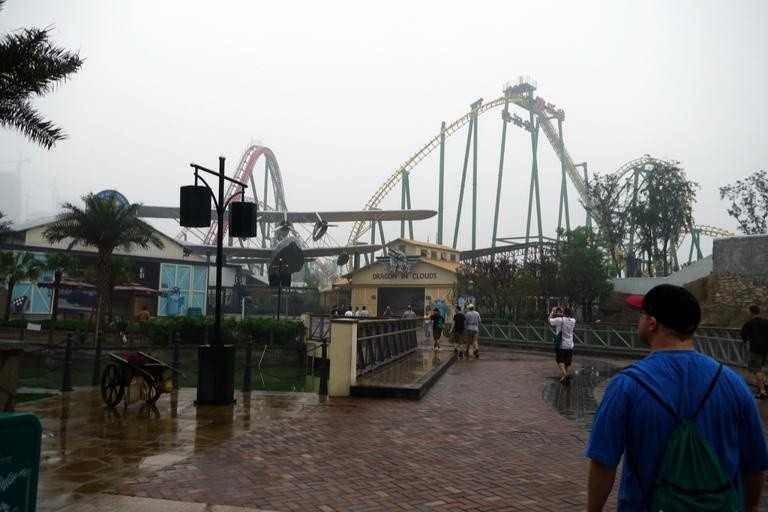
[197,345,236,403]
[1,411,42,512]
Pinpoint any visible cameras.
[556,308,560,311]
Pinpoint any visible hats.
[433,308,439,311]
[466,304,473,309]
[625,282,702,332]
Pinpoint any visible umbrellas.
[37,277,97,290]
[112,281,162,297]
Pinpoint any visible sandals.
[755,392,768,398]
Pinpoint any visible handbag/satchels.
[552,331,562,346]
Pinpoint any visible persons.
[383,306,394,316]
[139,306,150,323]
[402,305,416,318]
[585,284,768,512]
[332,304,369,317]
[465,304,481,358]
[548,307,576,382]
[424,310,431,337]
[449,306,467,357]
[430,308,444,350]
[739,306,768,398]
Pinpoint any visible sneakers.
[455,346,480,359]
[432,348,441,350]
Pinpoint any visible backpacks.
[616,362,743,512]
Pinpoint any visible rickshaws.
[101,350,186,409]
